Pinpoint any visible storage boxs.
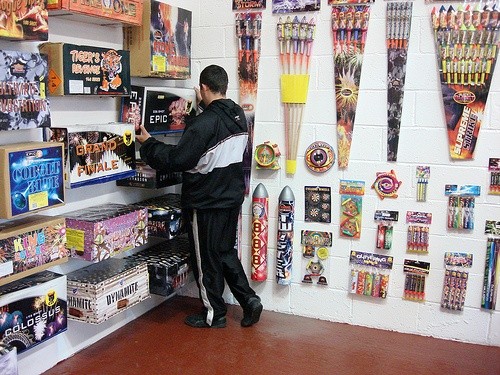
[0,0,200,374]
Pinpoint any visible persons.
[134,64,262,327]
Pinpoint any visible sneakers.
[185,312,226,327]
[241,298,263,327]
